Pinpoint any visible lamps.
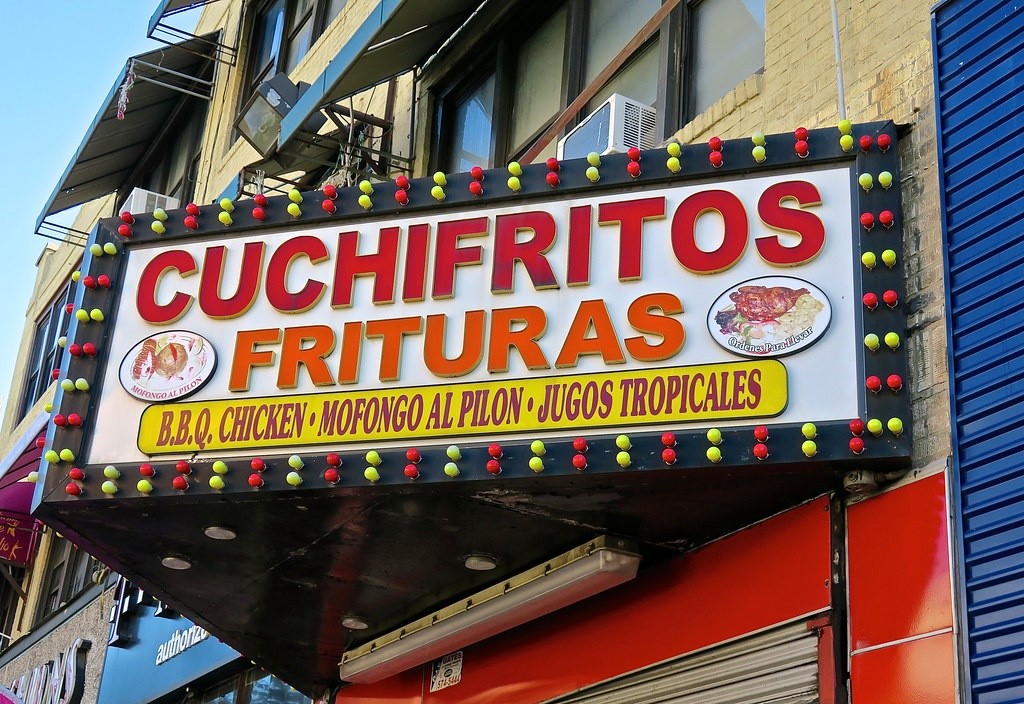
[337,533,643,686]
[233,73,391,177]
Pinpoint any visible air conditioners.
[556,91,656,161]
[119,187,178,216]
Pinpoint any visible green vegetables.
[737,325,753,345]
